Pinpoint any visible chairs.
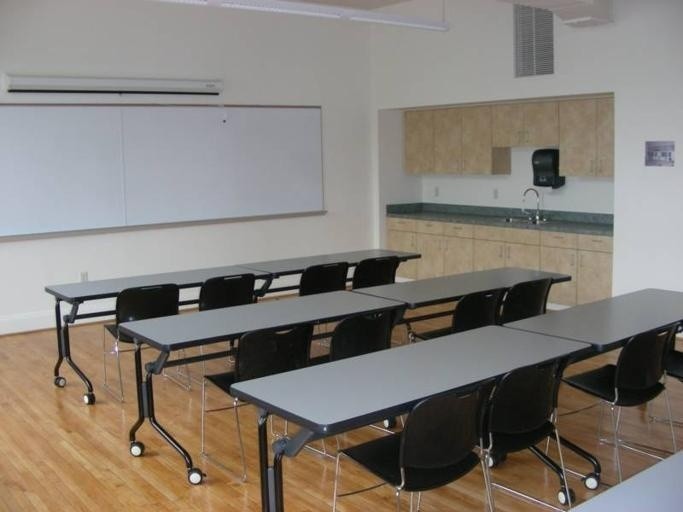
[546,320,683,489]
[409,277,554,344]
[201,305,407,482]
[332,355,571,512]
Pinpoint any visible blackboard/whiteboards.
[0,102,326,243]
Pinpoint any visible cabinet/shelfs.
[490,98,560,148]
[416,219,474,280]
[434,103,511,175]
[385,217,417,280]
[540,230,612,306]
[558,93,614,177]
[473,224,540,273]
[401,106,434,175]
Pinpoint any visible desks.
[44,265,273,405]
[235,248,421,347]
[231,325,596,512]
[116,291,407,484]
[351,267,572,345]
[503,289,682,489]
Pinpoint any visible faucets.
[522,188,540,220]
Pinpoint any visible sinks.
[479,217,549,226]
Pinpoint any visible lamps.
[172,0,453,34]
